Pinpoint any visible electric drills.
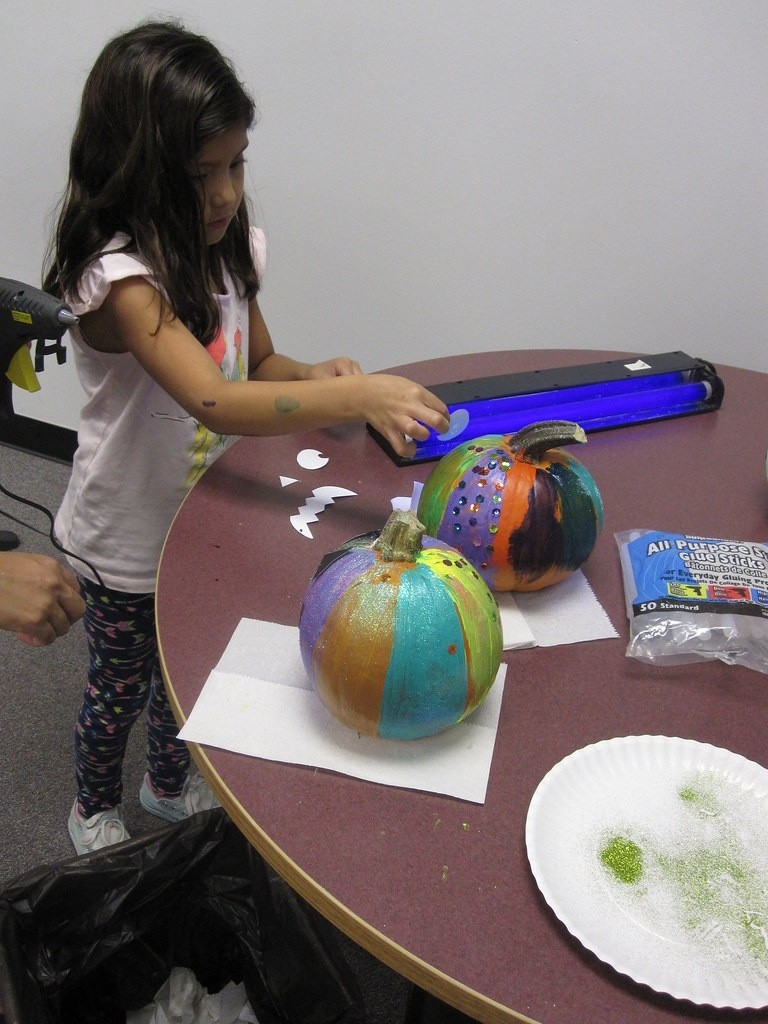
[0,276,155,608]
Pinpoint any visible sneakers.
[139,771,222,824]
[67,797,131,856]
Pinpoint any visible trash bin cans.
[0,807,374,1024]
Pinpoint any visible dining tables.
[149,346,768,1022]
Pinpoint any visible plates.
[525,735,768,1010]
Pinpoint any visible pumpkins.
[296,419,604,740]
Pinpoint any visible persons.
[1,535,88,649]
[49,19,454,865]
